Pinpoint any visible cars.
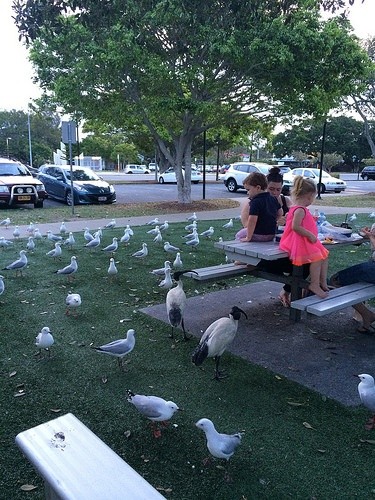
[359,165,375,181]
[191,161,347,196]
[148,162,160,172]
[156,166,203,184]
[36,164,117,206]
[124,164,151,174]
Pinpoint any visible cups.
[341,222,348,228]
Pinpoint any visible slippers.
[277,292,291,308]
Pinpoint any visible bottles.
[313,208,327,234]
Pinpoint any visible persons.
[241,167,311,308]
[329,222,375,332]
[234,172,283,265]
[279,175,329,299]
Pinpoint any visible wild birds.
[163,241,180,254]
[353,373,375,430]
[173,253,183,268]
[0,217,10,228]
[2,249,30,277]
[46,243,62,261]
[192,304,248,384]
[149,260,172,276]
[125,389,184,438]
[131,242,148,260]
[65,294,82,314]
[368,212,375,219]
[0,212,242,257]
[165,269,198,341]
[0,275,7,294]
[348,213,357,222]
[54,255,78,284]
[34,327,53,358]
[108,258,119,279]
[92,329,136,372]
[158,268,173,293]
[194,417,244,483]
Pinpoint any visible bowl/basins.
[343,233,352,238]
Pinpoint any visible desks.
[214,233,370,322]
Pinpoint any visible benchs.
[16,413,167,500]
[183,262,260,280]
[290,280,375,316]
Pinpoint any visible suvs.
[0,157,49,209]
